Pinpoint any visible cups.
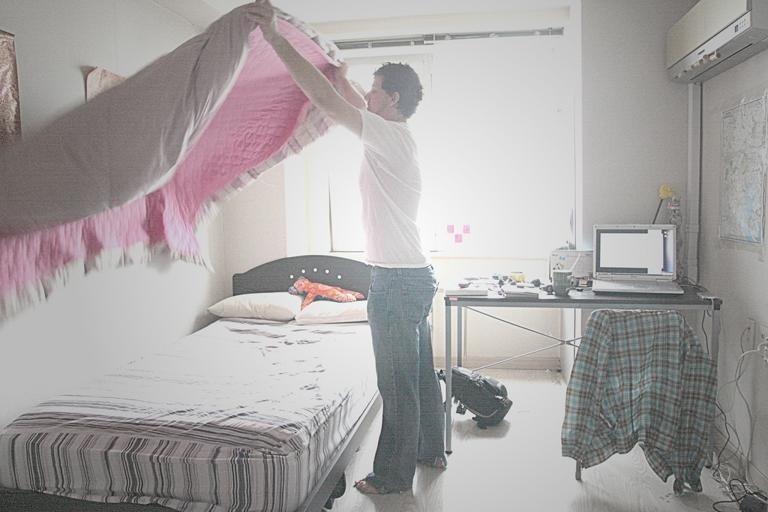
[552,271,577,296]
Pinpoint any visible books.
[502,286,539,300]
[445,287,489,296]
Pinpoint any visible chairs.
[562,308,717,490]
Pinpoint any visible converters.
[712,478,767,512]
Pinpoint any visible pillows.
[209,292,304,322]
[296,299,368,325]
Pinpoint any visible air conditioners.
[666,0,768,85]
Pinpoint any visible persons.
[246,0,447,496]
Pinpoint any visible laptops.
[590,222,685,297]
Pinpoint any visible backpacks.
[440,365,512,431]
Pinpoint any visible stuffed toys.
[289,276,365,311]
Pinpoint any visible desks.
[446,279,722,468]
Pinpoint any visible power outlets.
[757,323,768,358]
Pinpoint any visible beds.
[0,255,380,512]
